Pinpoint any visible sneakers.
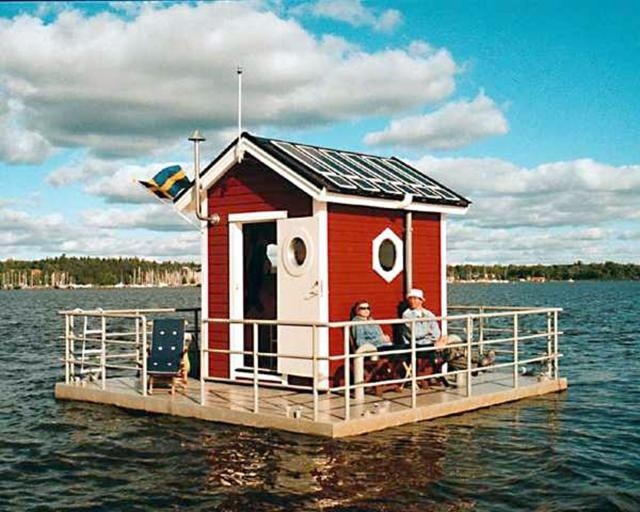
[478,348,496,373]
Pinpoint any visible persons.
[401,288,458,386]
[351,298,449,359]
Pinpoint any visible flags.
[135,164,193,202]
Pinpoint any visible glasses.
[358,306,371,310]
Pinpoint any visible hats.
[406,288,426,302]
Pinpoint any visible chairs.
[147,316,185,394]
[344,325,455,396]
